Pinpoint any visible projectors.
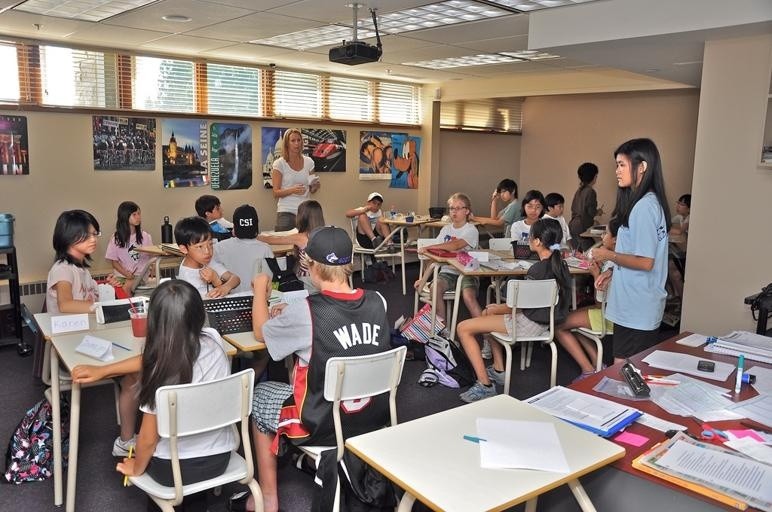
[329,41,378,66]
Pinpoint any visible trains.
[311,139,345,160]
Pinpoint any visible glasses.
[448,206,466,211]
[83,232,101,238]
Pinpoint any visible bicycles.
[97,149,150,168]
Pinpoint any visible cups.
[405,217,413,222]
[128,306,148,339]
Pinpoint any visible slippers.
[290,453,316,477]
[226,490,285,511]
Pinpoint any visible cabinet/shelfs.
[1,245,34,357]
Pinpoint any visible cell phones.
[698,361,714,372]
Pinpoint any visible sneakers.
[460,339,506,403]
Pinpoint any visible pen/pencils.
[735,354,744,394]
[112,343,130,351]
[463,435,487,443]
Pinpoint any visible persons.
[116,280,236,487]
[406,164,602,402]
[228,225,392,512]
[665,194,691,314]
[47,195,327,456]
[268,128,325,232]
[346,192,394,250]
[554,219,620,383]
[582,137,674,368]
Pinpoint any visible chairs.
[297,342,410,512]
[347,204,687,397]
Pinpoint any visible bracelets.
[612,252,617,266]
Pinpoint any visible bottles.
[161,216,173,243]
[736,373,758,385]
[517,231,529,245]
[390,205,396,220]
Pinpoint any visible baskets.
[202,296,270,335]
[511,240,541,260]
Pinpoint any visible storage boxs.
[0,212,16,248]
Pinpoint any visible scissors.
[691,416,728,443]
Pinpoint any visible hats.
[368,192,384,202]
[305,226,352,265]
[233,204,259,238]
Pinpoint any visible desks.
[32,225,310,511]
[536,327,772,511]
[342,391,628,512]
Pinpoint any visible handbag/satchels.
[419,335,476,386]
[5,391,70,484]
[390,326,425,360]
[365,262,394,285]
